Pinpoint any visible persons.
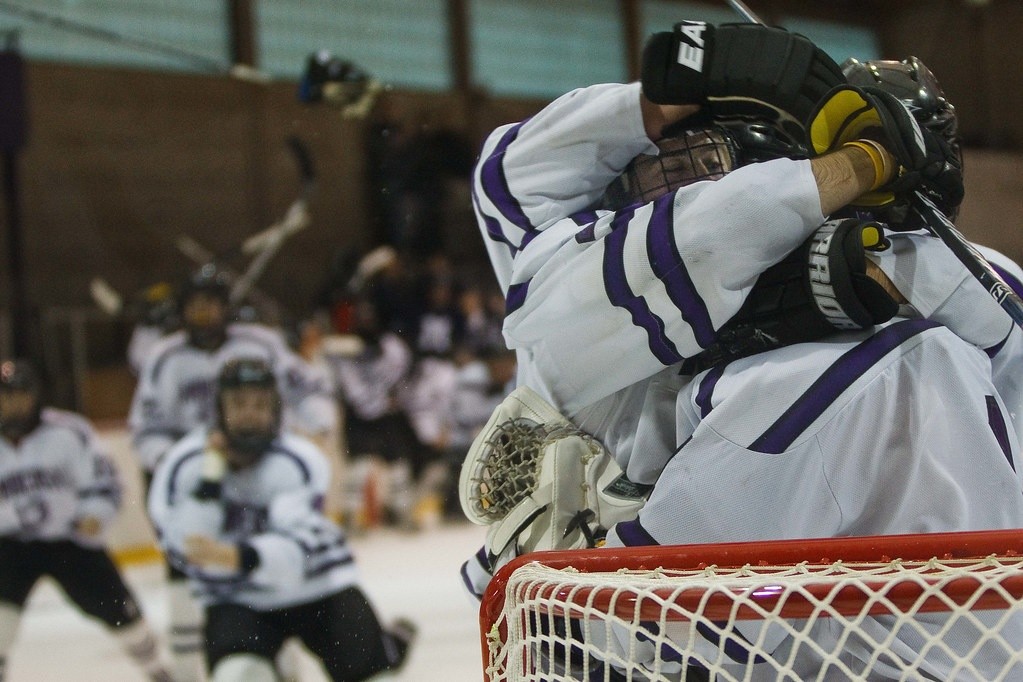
[148,349,417,680]
[0,1,1023,682]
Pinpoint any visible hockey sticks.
[725,0,1023,326]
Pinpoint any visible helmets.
[840,54,962,229]
[217,358,281,455]
[0,360,42,437]
[605,121,745,212]
[177,269,230,347]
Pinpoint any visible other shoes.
[386,619,415,670]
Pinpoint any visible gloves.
[804,84,964,231]
[641,20,848,146]
[703,218,899,366]
